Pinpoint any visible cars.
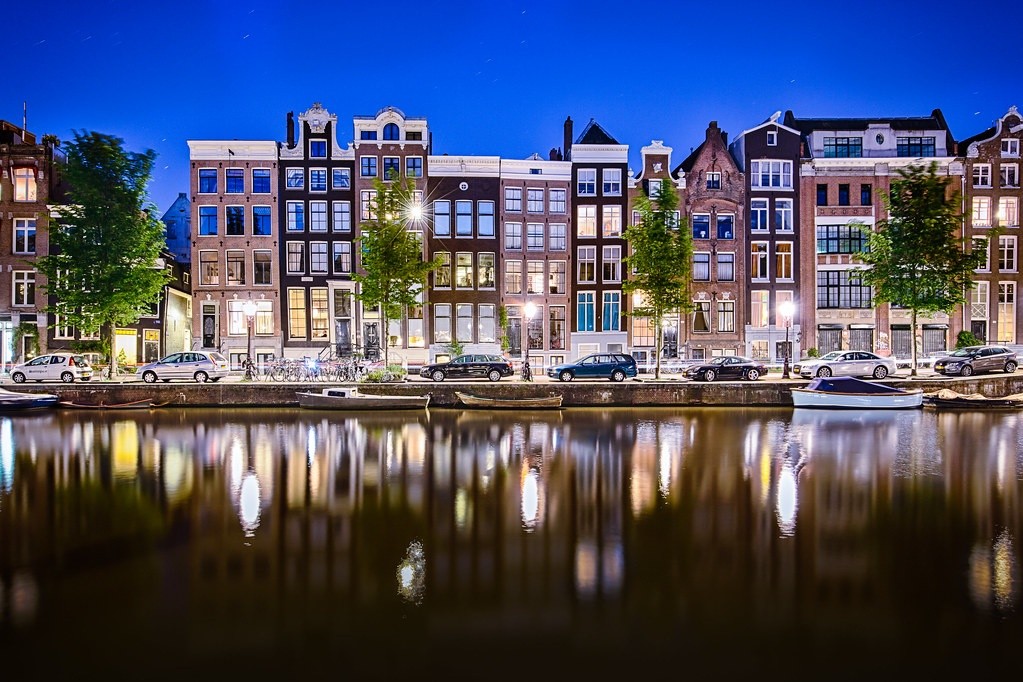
[9,353,94,383]
[792,350,897,379]
[682,356,768,382]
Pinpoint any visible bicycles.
[241,356,358,383]
[100,362,126,381]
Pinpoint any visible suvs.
[419,354,514,382]
[135,351,230,383]
[934,345,1018,376]
[546,353,639,382]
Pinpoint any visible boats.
[294,388,431,410]
[791,376,924,410]
[455,392,564,408]
[0,386,59,409]
[60,398,171,408]
[921,391,1023,408]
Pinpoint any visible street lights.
[521,300,535,379]
[778,301,794,379]
